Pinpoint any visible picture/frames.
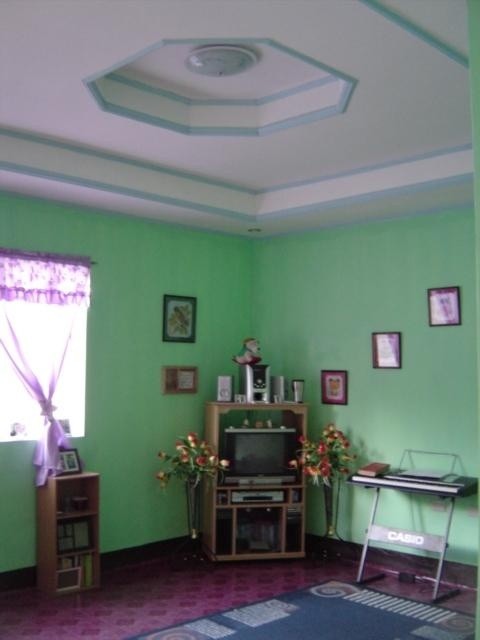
[371,331,401,369]
[161,365,199,395]
[162,294,197,344]
[58,448,83,474]
[320,369,348,406]
[426,285,462,327]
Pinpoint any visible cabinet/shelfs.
[344,447,479,604]
[200,400,307,562]
[36,472,102,596]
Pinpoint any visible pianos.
[344,468,477,496]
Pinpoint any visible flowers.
[288,423,354,488]
[155,431,232,490]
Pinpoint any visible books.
[357,463,389,478]
[57,553,94,591]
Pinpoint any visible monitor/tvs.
[224,428,298,484]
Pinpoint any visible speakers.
[217,375,232,402]
[270,375,285,402]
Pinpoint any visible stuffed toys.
[233,337,262,364]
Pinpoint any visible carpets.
[124,579,475,640]
